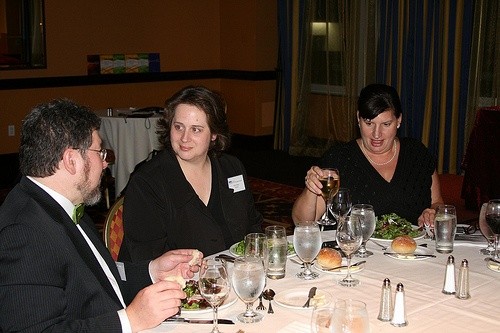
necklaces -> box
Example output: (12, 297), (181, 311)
(361, 139), (396, 164)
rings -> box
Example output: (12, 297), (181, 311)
(305, 179), (309, 182)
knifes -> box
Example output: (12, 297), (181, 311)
(302, 286), (317, 308)
(160, 317), (236, 325)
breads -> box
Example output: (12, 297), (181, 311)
(188, 250), (200, 266)
(316, 248), (342, 268)
(392, 235), (417, 253)
(160, 274), (187, 289)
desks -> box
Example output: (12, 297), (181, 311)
(460, 107), (500, 209)
(136, 231), (500, 333)
(99, 116), (168, 198)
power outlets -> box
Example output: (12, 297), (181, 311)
(8, 125), (15, 136)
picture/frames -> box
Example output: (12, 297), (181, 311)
(0, 0), (47, 69)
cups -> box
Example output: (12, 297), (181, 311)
(434, 205), (458, 254)
(243, 233), (267, 277)
(311, 298), (369, 333)
(263, 225), (287, 279)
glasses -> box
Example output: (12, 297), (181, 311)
(68, 146), (108, 162)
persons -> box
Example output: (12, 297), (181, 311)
(292, 85), (445, 232)
(123, 84), (263, 298)
(0, 99), (202, 333)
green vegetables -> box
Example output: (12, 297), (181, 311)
(369, 213), (421, 239)
(180, 278), (223, 309)
(233, 237), (295, 257)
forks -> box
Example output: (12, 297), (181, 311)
(256, 291), (265, 310)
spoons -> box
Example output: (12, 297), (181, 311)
(263, 287), (276, 314)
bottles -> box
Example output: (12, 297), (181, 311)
(456, 259), (472, 299)
(106, 108), (113, 117)
(377, 277), (394, 322)
(441, 254), (457, 295)
(389, 283), (409, 328)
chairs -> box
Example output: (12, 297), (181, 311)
(104, 196), (124, 264)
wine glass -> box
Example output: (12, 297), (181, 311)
(233, 256), (264, 324)
(294, 221), (323, 279)
(198, 257), (232, 333)
(316, 170), (378, 287)
(480, 199), (500, 273)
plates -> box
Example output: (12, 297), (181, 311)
(160, 275), (238, 313)
(384, 246), (433, 260)
(229, 237), (299, 261)
(273, 286), (332, 310)
(313, 258), (365, 274)
(368, 223), (426, 242)
